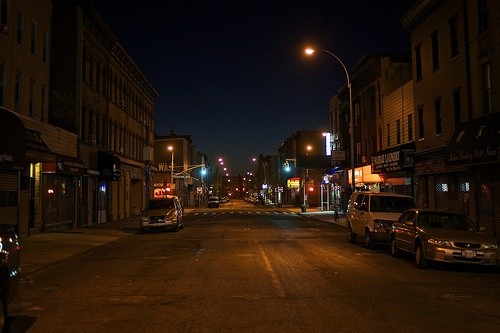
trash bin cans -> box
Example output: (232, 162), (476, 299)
(300, 205), (306, 212)
(278, 202), (283, 208)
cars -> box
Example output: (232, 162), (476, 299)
(0, 223), (25, 333)
(243, 193), (266, 205)
(206, 195), (230, 208)
(390, 208), (500, 270)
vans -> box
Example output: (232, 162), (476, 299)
(345, 191), (418, 250)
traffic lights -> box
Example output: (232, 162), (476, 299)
(200, 166), (207, 177)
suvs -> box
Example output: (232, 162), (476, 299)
(139, 196), (184, 234)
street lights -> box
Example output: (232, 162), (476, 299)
(252, 158), (267, 205)
(167, 145), (174, 195)
(304, 47), (356, 193)
(217, 167), (226, 197)
